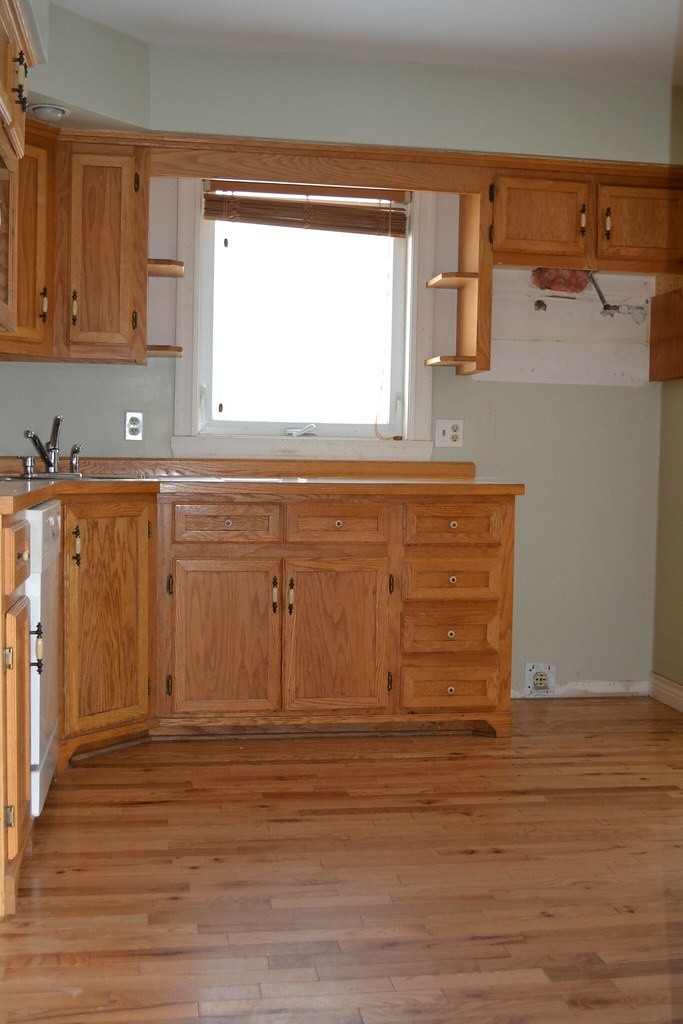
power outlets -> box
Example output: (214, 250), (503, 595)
(436, 419), (462, 446)
(526, 663), (555, 695)
(125, 411), (142, 440)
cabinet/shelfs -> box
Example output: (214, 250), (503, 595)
(0, 517), (32, 917)
(148, 258), (185, 357)
(0, 2), (36, 331)
(61, 494), (149, 771)
(491, 175), (683, 275)
(397, 496), (516, 738)
(0, 136), (148, 364)
(426, 273), (477, 366)
(156, 497), (388, 740)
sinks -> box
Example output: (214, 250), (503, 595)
(1, 475), (63, 481)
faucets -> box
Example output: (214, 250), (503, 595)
(22, 414), (63, 474)
